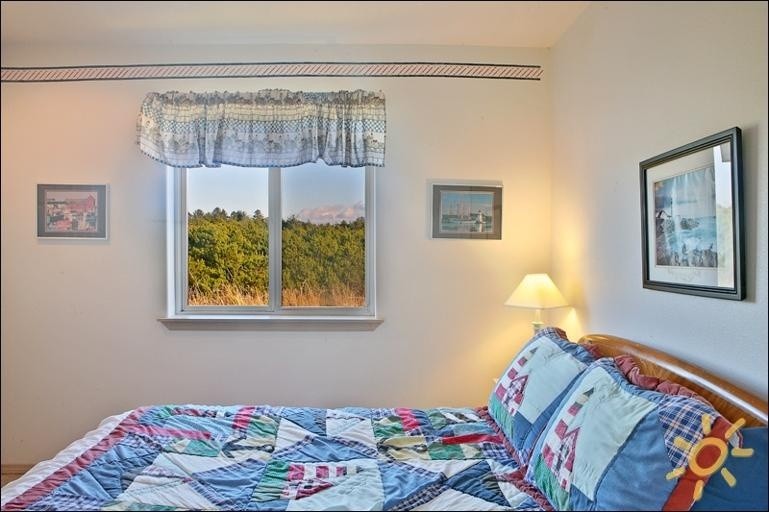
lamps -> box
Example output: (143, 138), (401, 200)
(504, 271), (572, 340)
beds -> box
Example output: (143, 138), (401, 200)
(0, 335), (769, 512)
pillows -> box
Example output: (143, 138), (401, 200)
(503, 354), (740, 511)
(478, 328), (606, 478)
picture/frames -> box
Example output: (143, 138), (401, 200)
(35, 183), (109, 238)
(433, 185), (503, 240)
(638, 127), (745, 302)
